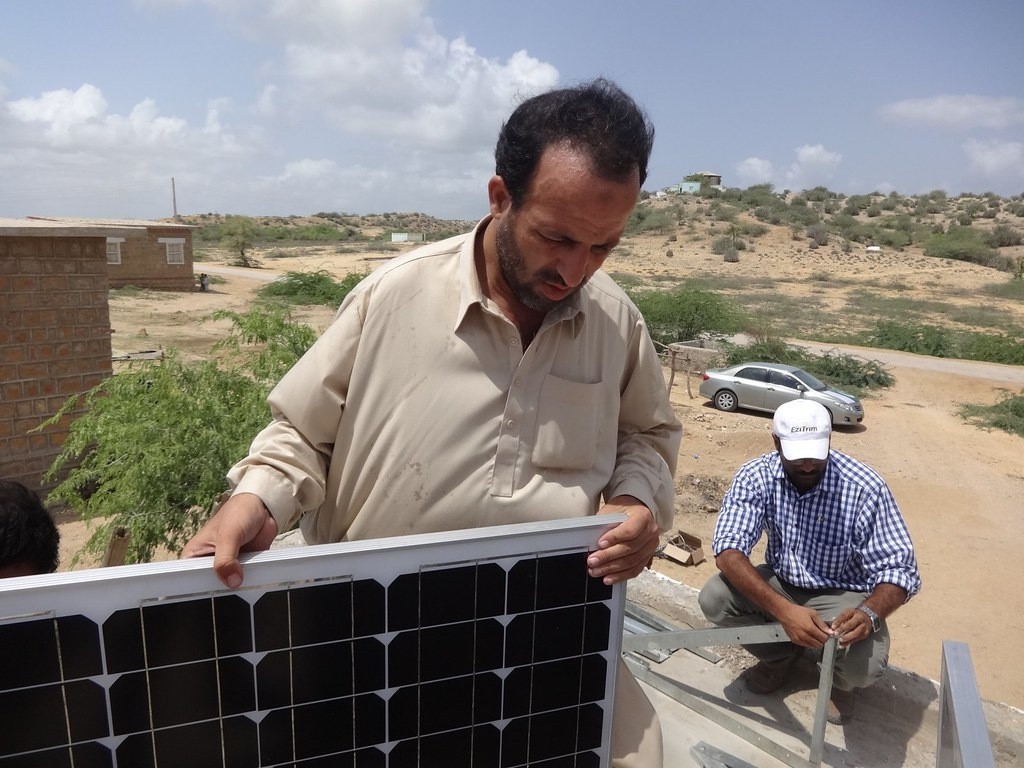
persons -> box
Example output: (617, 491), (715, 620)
(179, 84), (686, 767)
(198, 273), (209, 292)
(698, 398), (924, 723)
(0, 479), (60, 578)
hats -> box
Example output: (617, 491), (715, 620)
(773, 399), (832, 462)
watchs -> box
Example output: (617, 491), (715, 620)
(855, 604), (882, 634)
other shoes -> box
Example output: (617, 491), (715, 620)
(744, 641), (806, 694)
(826, 683), (857, 725)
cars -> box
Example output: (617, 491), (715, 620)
(698, 361), (866, 428)
